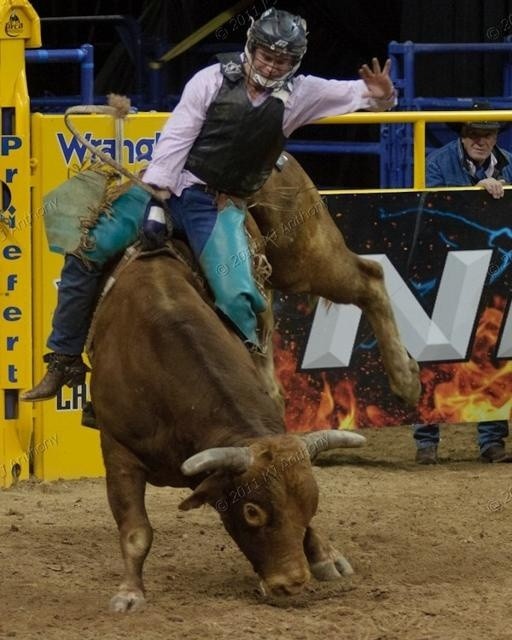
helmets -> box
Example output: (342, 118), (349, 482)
(241, 8), (308, 92)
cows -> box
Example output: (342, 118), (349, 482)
(85, 150), (422, 616)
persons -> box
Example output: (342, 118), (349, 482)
(424, 121), (512, 199)
(23, 9), (398, 401)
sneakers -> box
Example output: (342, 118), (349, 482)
(415, 441), (437, 465)
(478, 444), (509, 464)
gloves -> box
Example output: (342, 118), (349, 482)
(141, 200), (168, 252)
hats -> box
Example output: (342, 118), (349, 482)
(469, 103), (501, 129)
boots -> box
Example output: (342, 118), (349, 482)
(21, 355), (81, 400)
